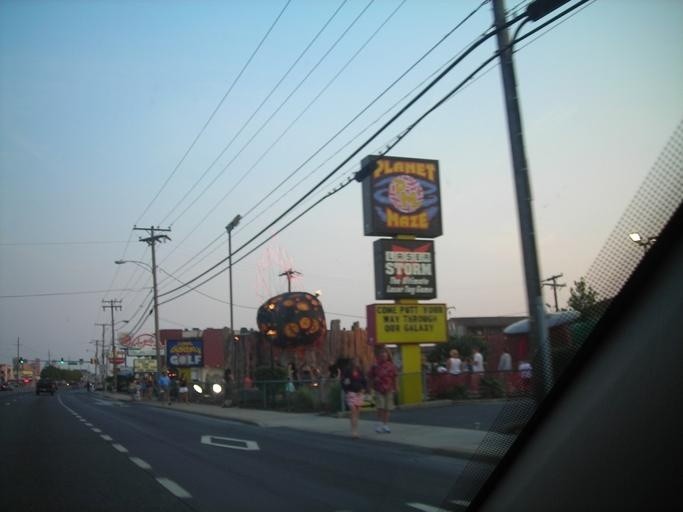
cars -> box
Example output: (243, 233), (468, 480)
(36, 379), (57, 396)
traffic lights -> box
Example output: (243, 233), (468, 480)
(61, 357), (63, 365)
(19, 356), (24, 364)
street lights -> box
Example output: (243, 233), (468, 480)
(114, 258), (162, 374)
(224, 214), (241, 387)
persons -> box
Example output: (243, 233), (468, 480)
(329, 344), (373, 438)
(364, 347), (399, 436)
(134, 365), (296, 410)
(422, 346), (532, 396)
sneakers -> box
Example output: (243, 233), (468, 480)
(376, 425), (391, 433)
